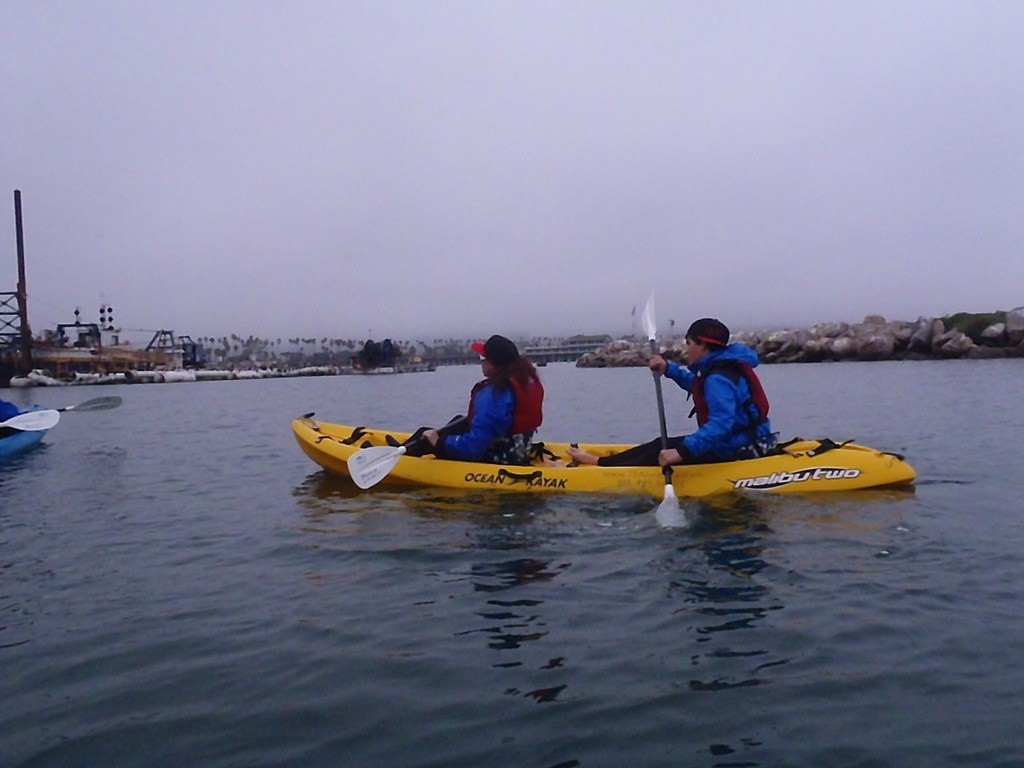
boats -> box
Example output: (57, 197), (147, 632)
(286, 409), (921, 491)
(366, 367), (396, 375)
(141, 329), (183, 370)
(0, 320), (167, 369)
(536, 358), (547, 367)
(0, 402), (55, 462)
(398, 361), (437, 373)
(10, 363), (343, 389)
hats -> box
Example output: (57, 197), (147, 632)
(472, 334), (519, 371)
(687, 318), (731, 352)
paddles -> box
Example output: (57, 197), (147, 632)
(0, 409), (60, 431)
(641, 303), (690, 530)
(346, 416), (467, 490)
(57, 395), (123, 413)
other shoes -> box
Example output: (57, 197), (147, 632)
(386, 435), (401, 448)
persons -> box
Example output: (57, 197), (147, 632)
(360, 334), (535, 465)
(543, 318), (770, 468)
